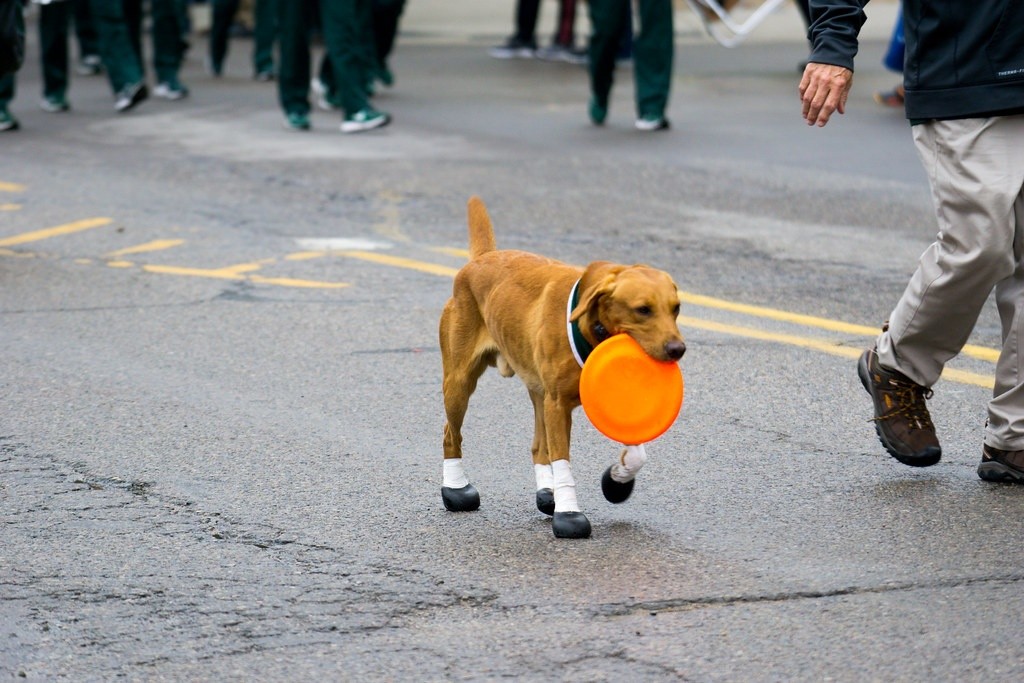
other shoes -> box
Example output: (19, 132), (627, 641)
(115, 84), (146, 112)
(590, 95), (607, 125)
(285, 111), (310, 129)
(378, 67), (394, 87)
(77, 61), (98, 77)
(546, 44), (591, 66)
(0, 107), (17, 132)
(488, 39), (533, 58)
(38, 91), (68, 112)
(873, 82), (906, 107)
(342, 104), (389, 133)
(637, 114), (669, 131)
(154, 79), (186, 101)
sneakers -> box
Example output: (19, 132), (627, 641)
(858, 346), (942, 468)
(975, 440), (1024, 485)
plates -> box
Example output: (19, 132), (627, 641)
(579, 334), (685, 448)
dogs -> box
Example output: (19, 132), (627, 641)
(439, 198), (685, 540)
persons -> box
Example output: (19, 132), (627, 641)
(871, 6), (905, 112)
(589, 0), (673, 130)
(562, 0), (634, 66)
(491, 0), (575, 60)
(799, 0), (1024, 483)
(1, 1), (406, 133)
